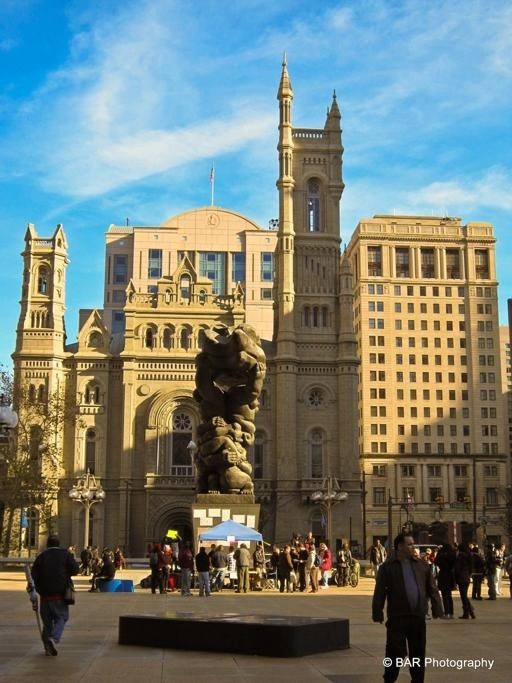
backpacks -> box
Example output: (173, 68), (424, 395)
(315, 554), (321, 567)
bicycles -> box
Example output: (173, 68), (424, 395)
(334, 563), (358, 587)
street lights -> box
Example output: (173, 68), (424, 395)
(0, 407), (19, 464)
(311, 474), (348, 544)
(69, 468), (106, 550)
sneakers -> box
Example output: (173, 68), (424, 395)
(47, 639), (58, 656)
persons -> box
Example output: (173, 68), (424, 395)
(67, 530), (355, 593)
(411, 540), (511, 619)
(369, 537), (386, 575)
(27, 533), (77, 658)
(370, 531), (441, 683)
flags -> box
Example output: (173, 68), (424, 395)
(21, 509), (29, 528)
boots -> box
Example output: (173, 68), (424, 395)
(459, 607), (475, 619)
